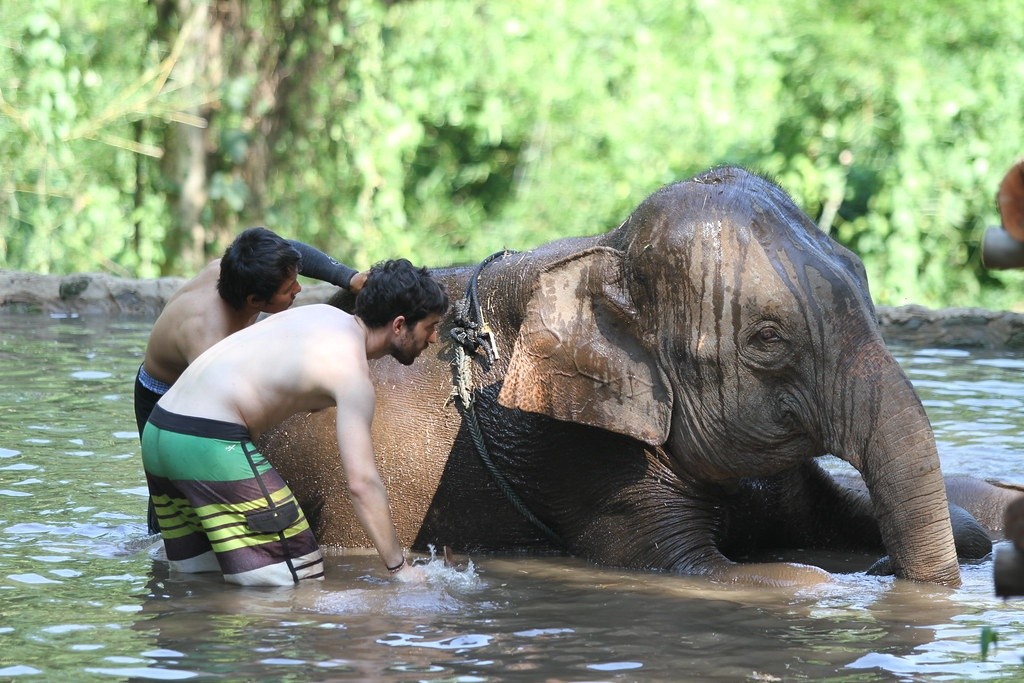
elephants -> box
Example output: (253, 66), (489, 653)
(243, 165), (1022, 604)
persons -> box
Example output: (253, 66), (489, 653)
(134, 228), (380, 537)
(140, 257), (451, 591)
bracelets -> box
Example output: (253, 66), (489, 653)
(386, 555), (408, 573)
